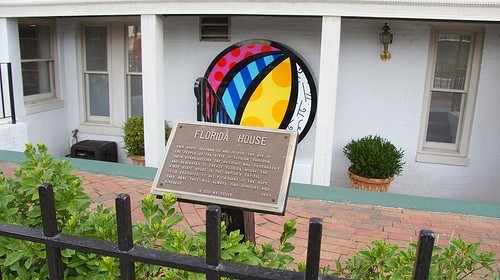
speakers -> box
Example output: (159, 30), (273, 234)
(71, 140), (118, 162)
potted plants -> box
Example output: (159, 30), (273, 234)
(119, 113), (173, 166)
(342, 134), (405, 193)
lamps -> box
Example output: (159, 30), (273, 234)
(378, 22), (393, 63)
